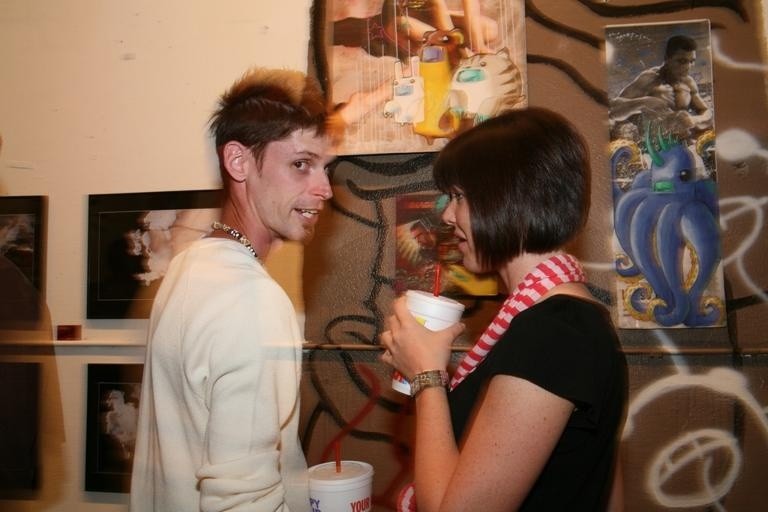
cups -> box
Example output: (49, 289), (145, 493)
(390, 290), (464, 397)
(305, 461), (375, 510)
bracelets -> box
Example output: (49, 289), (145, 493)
(409, 369), (450, 396)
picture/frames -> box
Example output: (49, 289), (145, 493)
(82, 360), (145, 496)
(85, 187), (223, 321)
(0, 193), (51, 330)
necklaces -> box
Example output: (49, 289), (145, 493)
(212, 220), (260, 261)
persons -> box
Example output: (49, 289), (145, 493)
(410, 219), (438, 262)
(128, 63), (340, 512)
(379, 105), (630, 512)
(608, 34), (713, 147)
